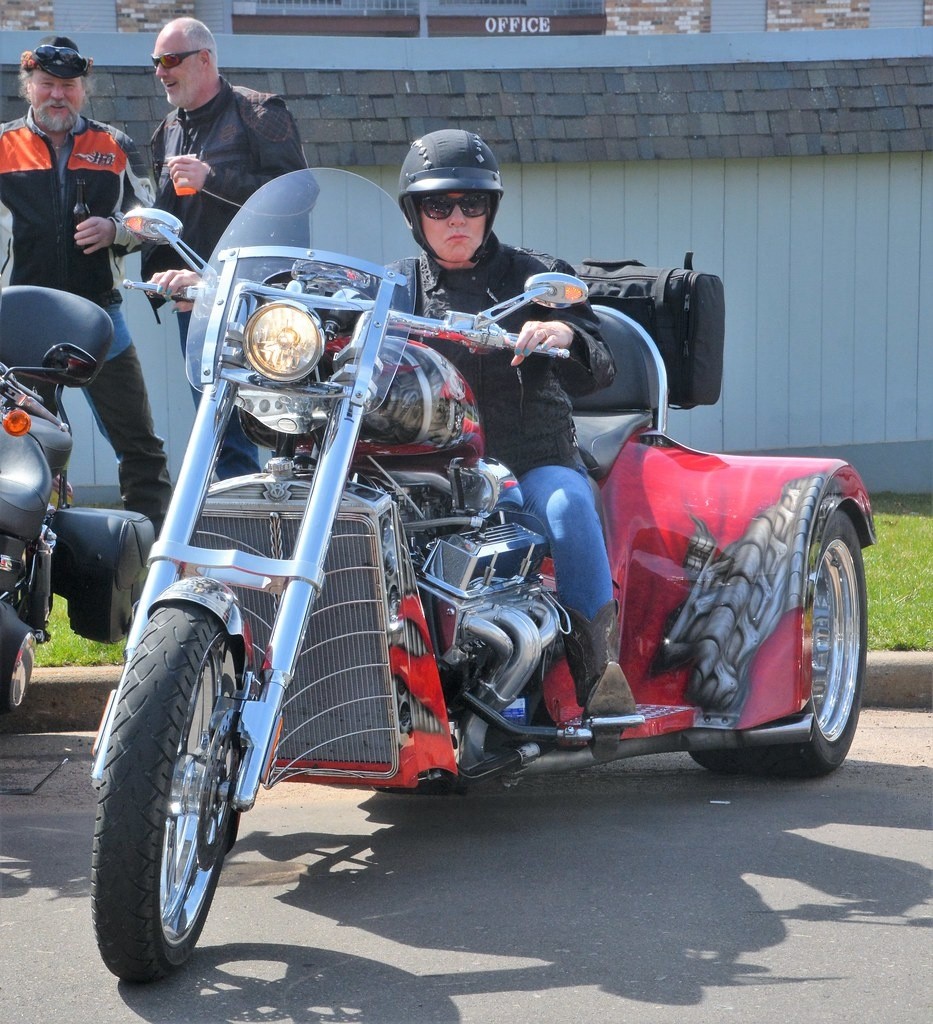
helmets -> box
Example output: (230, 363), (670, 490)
(398, 129), (504, 263)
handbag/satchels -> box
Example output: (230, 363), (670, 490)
(569, 251), (725, 410)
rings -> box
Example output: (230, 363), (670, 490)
(541, 329), (548, 338)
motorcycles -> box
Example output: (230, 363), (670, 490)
(0, 281), (161, 738)
(84, 166), (878, 988)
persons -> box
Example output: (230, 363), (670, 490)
(0, 34), (170, 542)
(141, 17), (319, 481)
(147, 130), (641, 762)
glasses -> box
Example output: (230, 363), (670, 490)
(150, 48), (211, 69)
(418, 193), (491, 219)
(33, 44), (83, 64)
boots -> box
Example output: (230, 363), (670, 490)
(555, 598), (637, 762)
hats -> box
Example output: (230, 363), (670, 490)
(24, 36), (89, 79)
